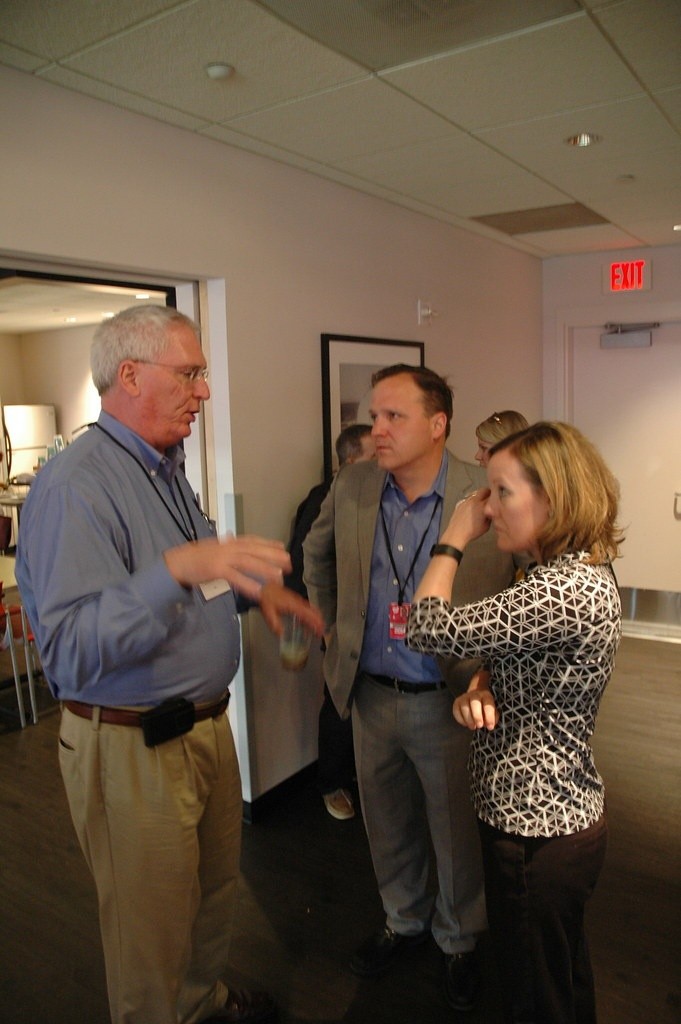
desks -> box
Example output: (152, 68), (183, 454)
(0, 491), (27, 561)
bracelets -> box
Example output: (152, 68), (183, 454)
(430, 543), (464, 567)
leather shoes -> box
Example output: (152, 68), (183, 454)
(360, 925), (429, 973)
(442, 947), (484, 1013)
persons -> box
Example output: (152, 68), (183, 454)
(15, 303), (326, 1024)
(281, 423), (376, 820)
(476, 410), (529, 469)
(302, 362), (534, 1009)
(404, 418), (623, 1023)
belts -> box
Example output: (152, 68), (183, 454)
(369, 674), (448, 692)
(63, 692), (232, 728)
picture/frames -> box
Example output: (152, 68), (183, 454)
(321, 332), (426, 483)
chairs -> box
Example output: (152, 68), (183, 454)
(0, 604), (40, 730)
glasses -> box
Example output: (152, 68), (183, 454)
(135, 359), (210, 384)
(488, 411), (506, 431)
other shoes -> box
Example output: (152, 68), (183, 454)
(321, 786), (356, 821)
(350, 777), (359, 783)
(191, 988), (282, 1024)
